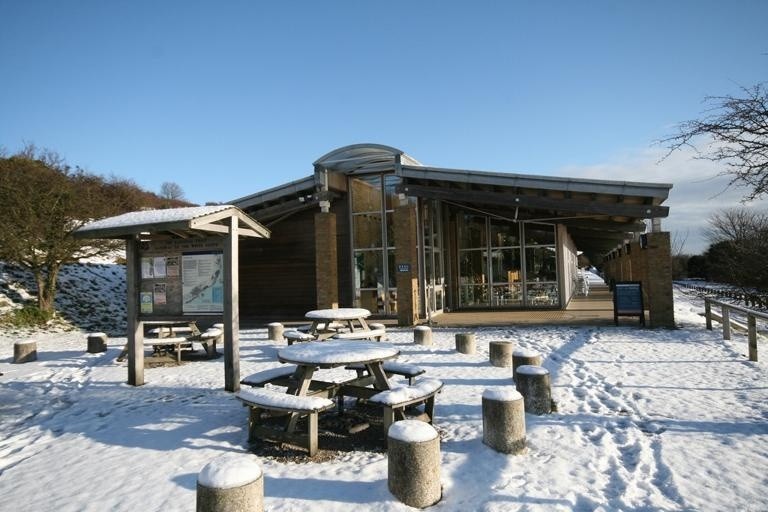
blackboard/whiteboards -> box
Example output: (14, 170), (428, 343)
(613, 281), (644, 316)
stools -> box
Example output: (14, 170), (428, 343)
(387, 419), (442, 507)
(489, 340), (513, 368)
(512, 350), (540, 383)
(13, 339), (38, 363)
(481, 388), (527, 455)
(413, 327), (433, 346)
(455, 333), (476, 354)
(267, 323), (284, 341)
(87, 333), (108, 352)
(193, 452), (264, 511)
(516, 365), (550, 416)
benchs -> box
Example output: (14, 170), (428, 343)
(238, 364), (320, 389)
(345, 360), (426, 386)
(333, 328), (385, 341)
(143, 336), (187, 365)
(369, 377), (445, 435)
(149, 326), (193, 338)
(201, 323), (224, 356)
(234, 387), (336, 456)
(297, 323), (344, 333)
(369, 323), (386, 342)
(343, 322), (386, 330)
(283, 329), (315, 345)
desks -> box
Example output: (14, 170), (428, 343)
(278, 340), (408, 437)
(305, 308), (376, 341)
